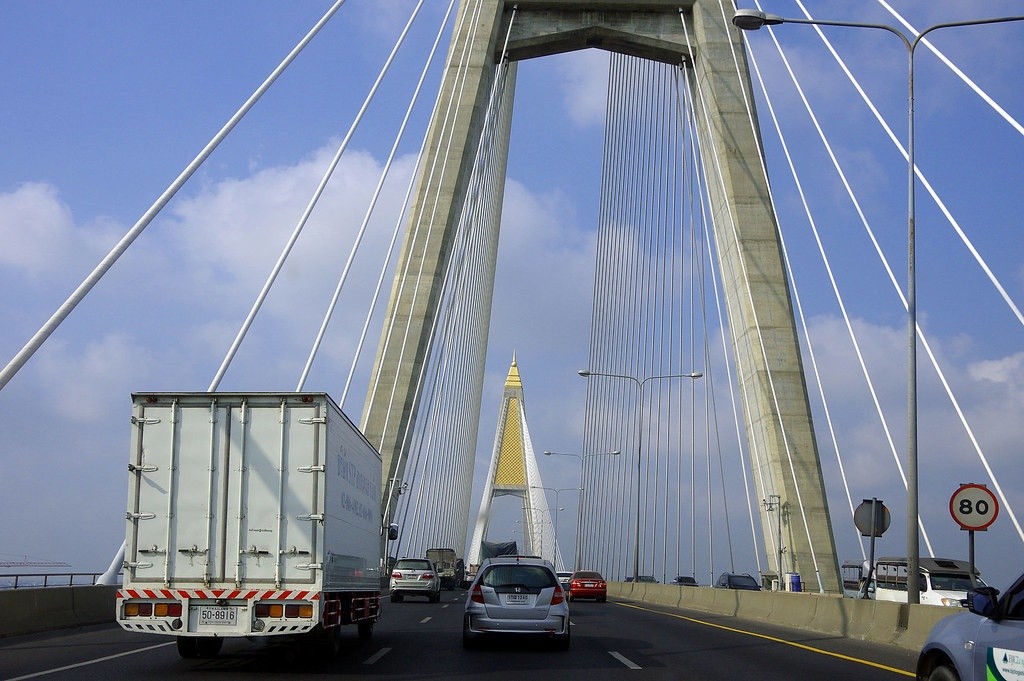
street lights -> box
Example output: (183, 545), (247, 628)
(521, 506), (564, 565)
(575, 369), (703, 581)
(530, 486), (583, 567)
(542, 449), (621, 572)
(731, 6), (1024, 604)
(765, 495), (785, 589)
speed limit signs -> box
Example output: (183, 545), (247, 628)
(949, 484), (1000, 530)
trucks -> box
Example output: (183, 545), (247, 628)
(840, 553), (992, 611)
(425, 547), (457, 592)
(480, 539), (516, 573)
(110, 385), (402, 661)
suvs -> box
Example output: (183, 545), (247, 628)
(712, 573), (762, 593)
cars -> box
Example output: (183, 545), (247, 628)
(567, 569), (607, 603)
(555, 570), (573, 597)
(624, 576), (659, 583)
(461, 555), (571, 651)
(673, 576), (697, 587)
(915, 571), (1024, 681)
(390, 556), (441, 602)
